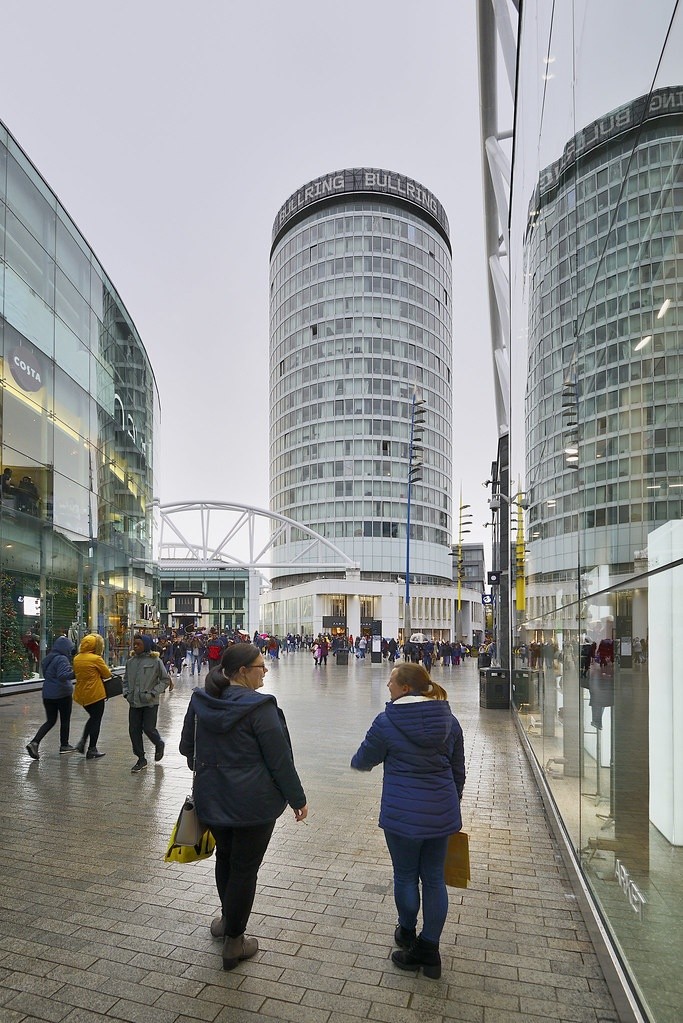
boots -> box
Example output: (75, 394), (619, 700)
(223, 929), (260, 970)
(210, 910), (225, 937)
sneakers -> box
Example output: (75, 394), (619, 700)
(26, 742), (40, 759)
(132, 758), (147, 772)
(155, 741), (164, 761)
(59, 744), (74, 753)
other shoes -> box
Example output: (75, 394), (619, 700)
(86, 746), (106, 759)
(74, 746), (86, 758)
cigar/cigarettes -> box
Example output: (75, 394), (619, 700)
(301, 819), (308, 825)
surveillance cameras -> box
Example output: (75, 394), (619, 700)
(520, 499), (529, 510)
(489, 501), (500, 512)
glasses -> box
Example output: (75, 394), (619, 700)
(248, 664), (265, 668)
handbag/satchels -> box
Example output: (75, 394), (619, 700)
(103, 673), (123, 698)
(262, 647), (265, 652)
(163, 816), (216, 864)
(174, 801), (208, 845)
(443, 832), (470, 889)
(193, 648), (199, 656)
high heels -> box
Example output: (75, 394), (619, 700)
(394, 924), (417, 949)
(391, 936), (441, 979)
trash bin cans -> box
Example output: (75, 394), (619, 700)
(336, 650), (349, 665)
(511, 667), (544, 715)
(480, 666), (510, 709)
(478, 652), (491, 669)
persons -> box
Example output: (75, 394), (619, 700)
(0, 467), (15, 489)
(23, 631), (40, 673)
(281, 633), (401, 665)
(26, 636), (74, 759)
(253, 630), (282, 662)
(520, 637), (648, 677)
(122, 634), (170, 772)
(349, 662), (467, 980)
(149, 624), (252, 678)
(488, 640), (496, 657)
(19, 476), (37, 512)
(402, 637), (480, 675)
(72, 633), (112, 759)
(179, 641), (308, 972)
